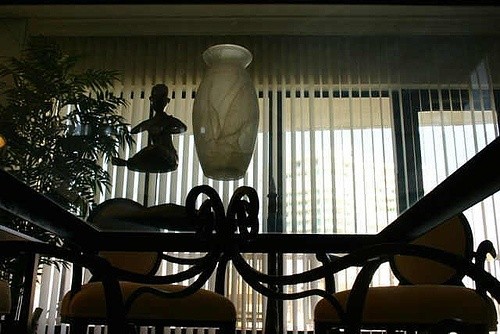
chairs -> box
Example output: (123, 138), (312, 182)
(61, 198), (236, 334)
(313, 211), (500, 334)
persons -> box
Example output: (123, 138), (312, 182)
(127, 81), (189, 174)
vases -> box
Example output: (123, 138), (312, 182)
(192, 44), (260, 181)
